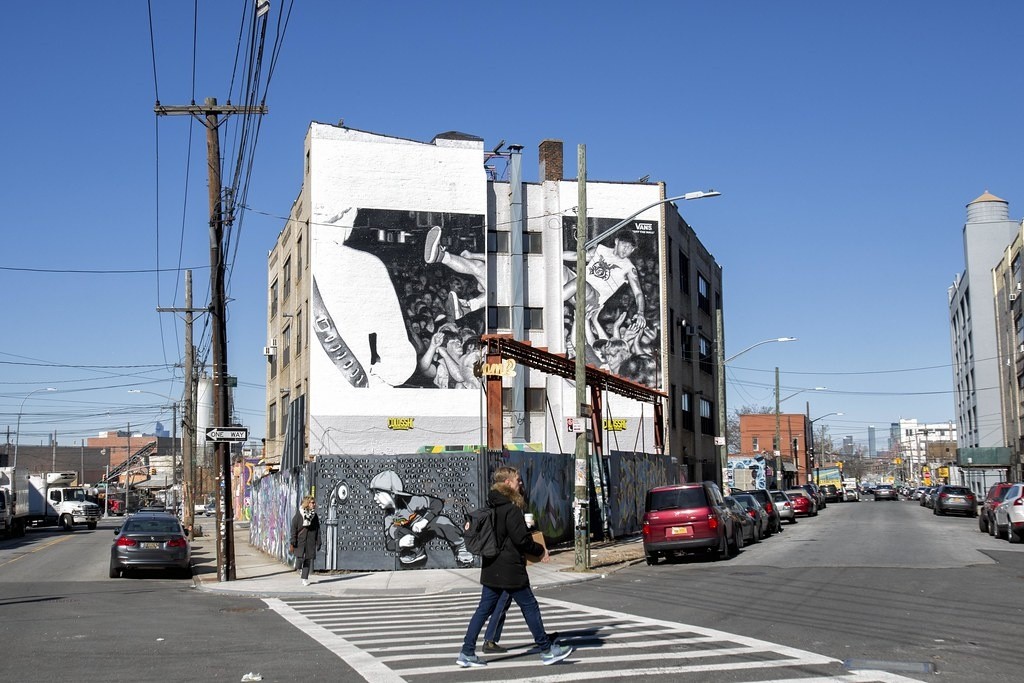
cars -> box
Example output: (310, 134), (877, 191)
(724, 484), (858, 548)
(204, 503), (216, 517)
(933, 485), (978, 518)
(108, 505), (191, 579)
(642, 481), (742, 566)
(861, 483), (938, 509)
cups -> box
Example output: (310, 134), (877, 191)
(524, 513), (533, 528)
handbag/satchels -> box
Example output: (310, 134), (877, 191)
(532, 531), (550, 563)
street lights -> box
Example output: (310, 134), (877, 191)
(573, 190), (720, 569)
(775, 388), (828, 491)
(127, 391), (185, 523)
(807, 413), (845, 485)
(822, 437), (854, 468)
(11, 388), (58, 516)
(717, 338), (797, 497)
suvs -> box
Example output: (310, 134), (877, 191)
(977, 482), (1024, 544)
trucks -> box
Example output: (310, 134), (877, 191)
(0, 466), (126, 541)
(166, 500), (207, 515)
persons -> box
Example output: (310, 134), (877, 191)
(290, 496), (322, 586)
(456, 467), (573, 667)
(311, 204), (663, 391)
(139, 498), (144, 506)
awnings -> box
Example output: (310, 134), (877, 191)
(782, 462), (798, 471)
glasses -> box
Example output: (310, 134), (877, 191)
(309, 502), (315, 504)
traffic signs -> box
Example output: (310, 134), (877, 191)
(580, 404), (593, 418)
(206, 427), (248, 442)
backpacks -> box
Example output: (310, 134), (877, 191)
(464, 505), (500, 556)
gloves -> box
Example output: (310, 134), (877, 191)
(525, 513), (536, 529)
(290, 544), (294, 553)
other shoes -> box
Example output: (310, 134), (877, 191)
(303, 578), (310, 586)
(300, 569), (303, 574)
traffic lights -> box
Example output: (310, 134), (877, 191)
(149, 469), (156, 476)
(103, 474), (106, 481)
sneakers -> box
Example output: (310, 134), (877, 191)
(456, 653), (487, 667)
(540, 644), (573, 665)
(547, 632), (559, 644)
(483, 641), (508, 653)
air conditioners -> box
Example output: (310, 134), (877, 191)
(264, 347), (276, 356)
(1009, 294), (1016, 301)
